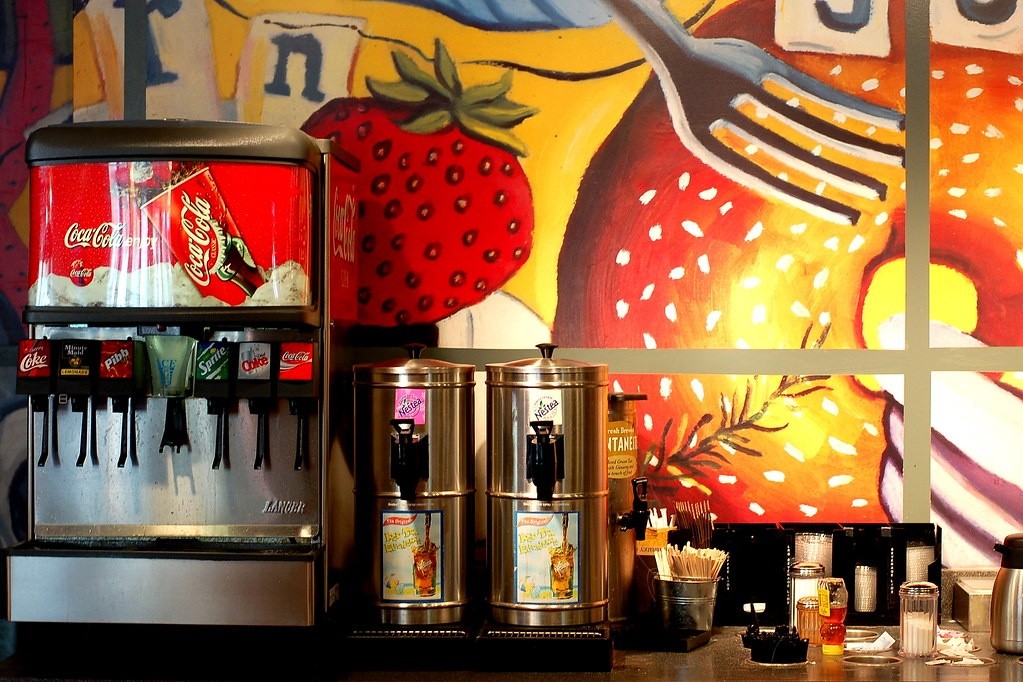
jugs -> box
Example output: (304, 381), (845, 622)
(989, 533), (1023, 655)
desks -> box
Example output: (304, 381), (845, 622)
(350, 625), (1023, 682)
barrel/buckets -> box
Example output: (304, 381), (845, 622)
(608, 395), (650, 628)
(365, 342), (477, 625)
(485, 343), (609, 627)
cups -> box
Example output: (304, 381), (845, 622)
(654, 576), (720, 632)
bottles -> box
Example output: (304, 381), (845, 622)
(788, 560), (827, 634)
(796, 596), (822, 647)
(898, 581), (940, 658)
(817, 577), (848, 656)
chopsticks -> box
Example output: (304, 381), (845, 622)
(654, 541), (729, 582)
